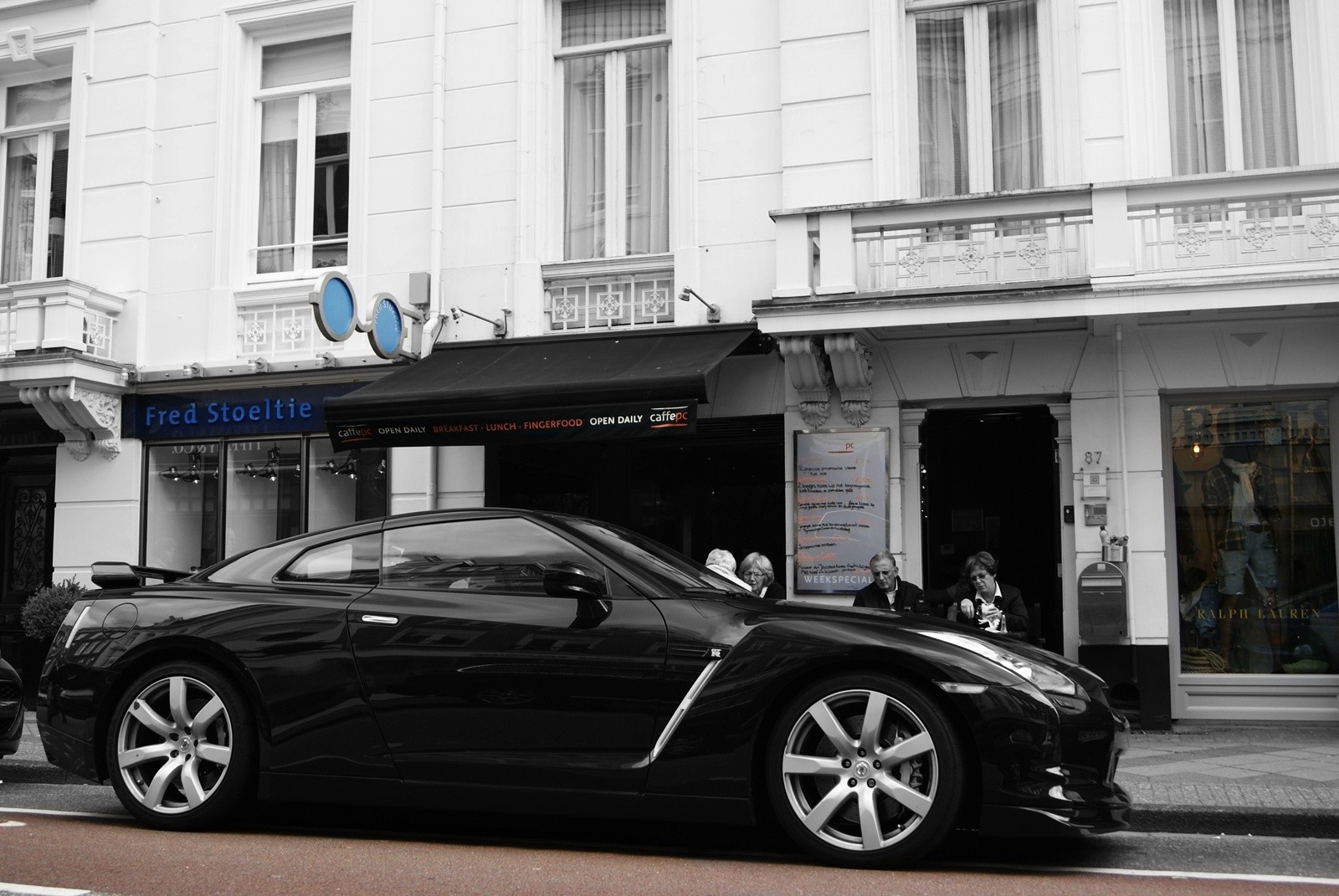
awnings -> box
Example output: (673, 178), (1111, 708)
(321, 322), (763, 455)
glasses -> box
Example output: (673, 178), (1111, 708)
(743, 572), (765, 579)
(970, 571), (988, 581)
(873, 570), (890, 576)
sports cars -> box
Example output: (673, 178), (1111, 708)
(33, 506), (1134, 867)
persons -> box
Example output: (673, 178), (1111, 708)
(1200, 457), (1289, 675)
(737, 551), (787, 599)
(954, 550), (1032, 635)
(698, 548), (753, 595)
(848, 551), (926, 613)
